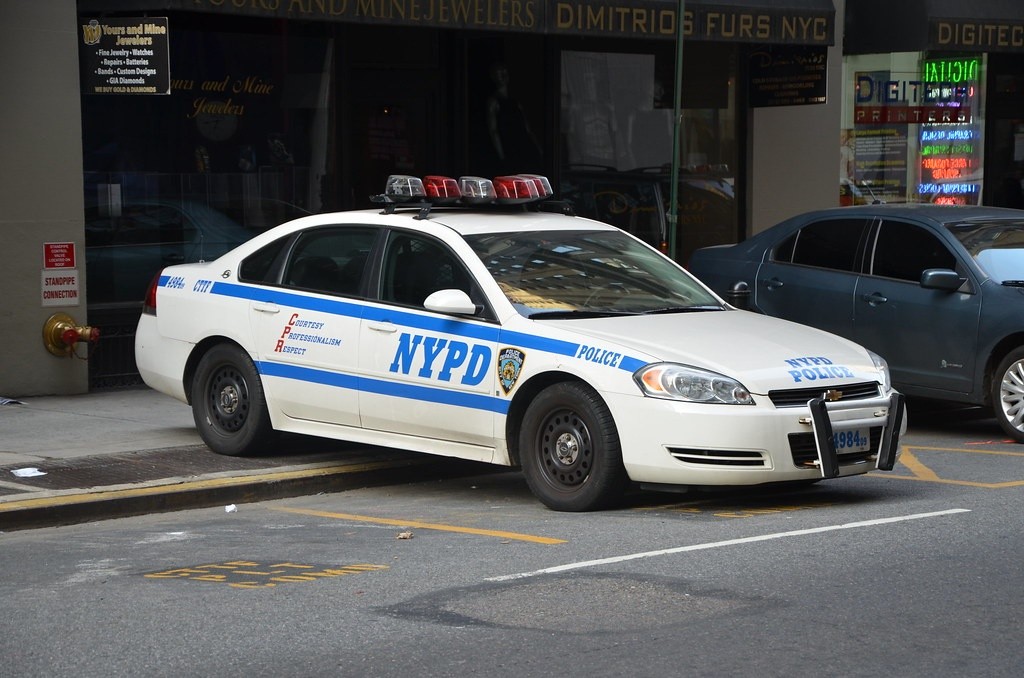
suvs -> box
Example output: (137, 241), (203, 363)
(549, 163), (692, 251)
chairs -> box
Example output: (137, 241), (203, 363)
(288, 252), (369, 296)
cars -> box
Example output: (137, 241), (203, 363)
(85, 195), (371, 327)
(133, 173), (909, 513)
(689, 204), (1023, 445)
